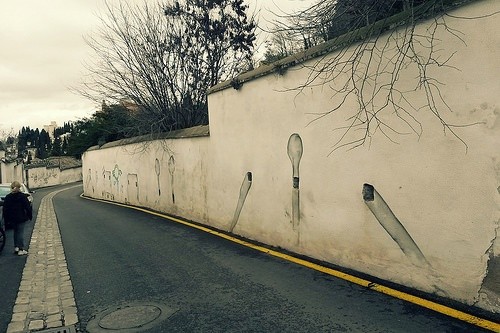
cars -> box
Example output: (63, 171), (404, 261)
(0, 182), (35, 208)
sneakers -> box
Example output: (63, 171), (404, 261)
(18, 249), (27, 255)
(14, 246), (19, 252)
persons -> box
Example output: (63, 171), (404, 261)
(2, 180), (33, 256)
